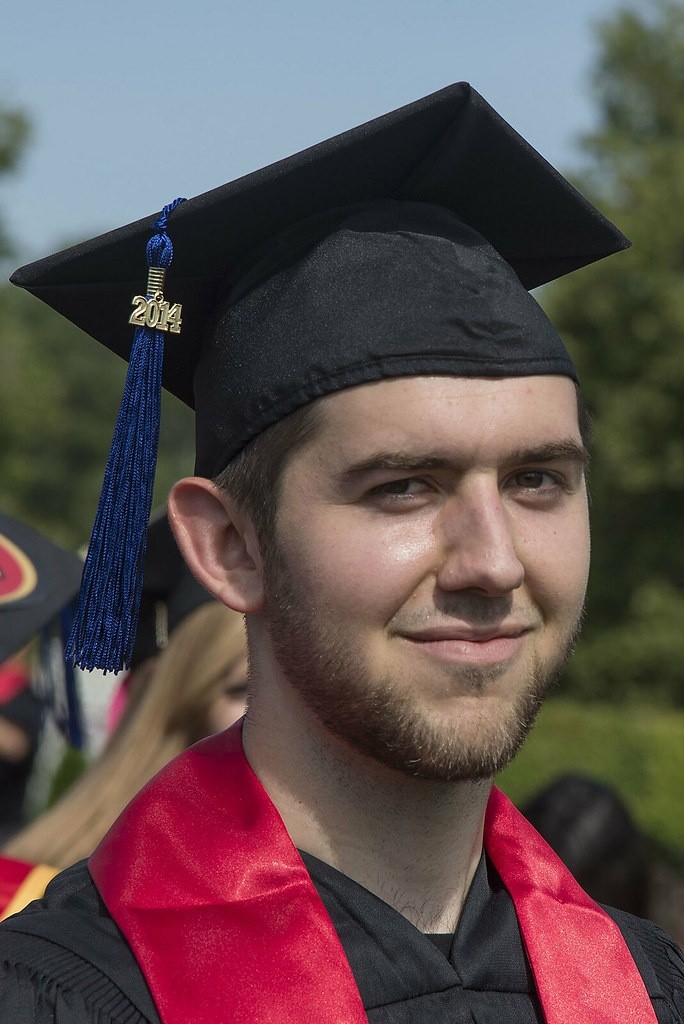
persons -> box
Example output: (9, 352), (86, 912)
(0, 509), (249, 919)
(0, 78), (683, 1024)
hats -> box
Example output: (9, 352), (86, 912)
(38, 511), (220, 749)
(0, 514), (87, 672)
(9, 82), (634, 676)
